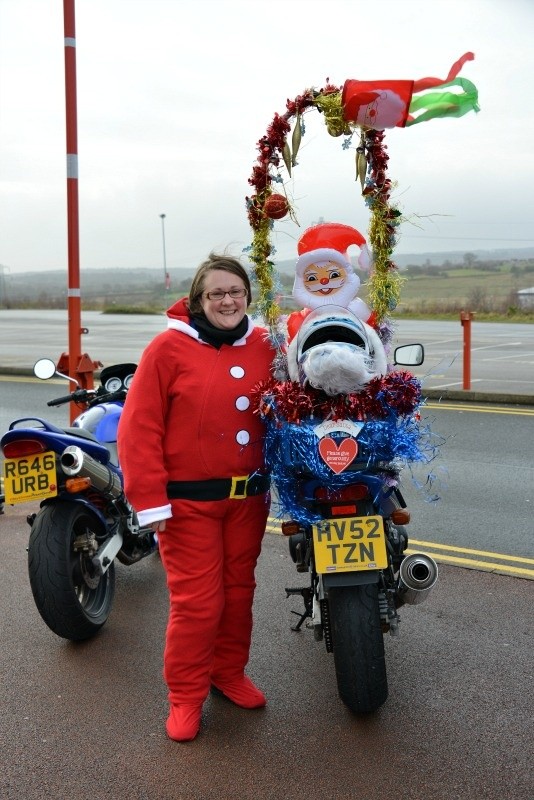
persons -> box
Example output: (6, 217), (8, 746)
(117, 253), (277, 741)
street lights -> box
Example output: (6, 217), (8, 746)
(160, 213), (168, 304)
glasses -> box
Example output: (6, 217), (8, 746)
(201, 289), (249, 300)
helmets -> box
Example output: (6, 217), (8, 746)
(296, 315), (377, 390)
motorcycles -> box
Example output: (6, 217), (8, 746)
(0, 357), (160, 641)
(281, 343), (439, 718)
